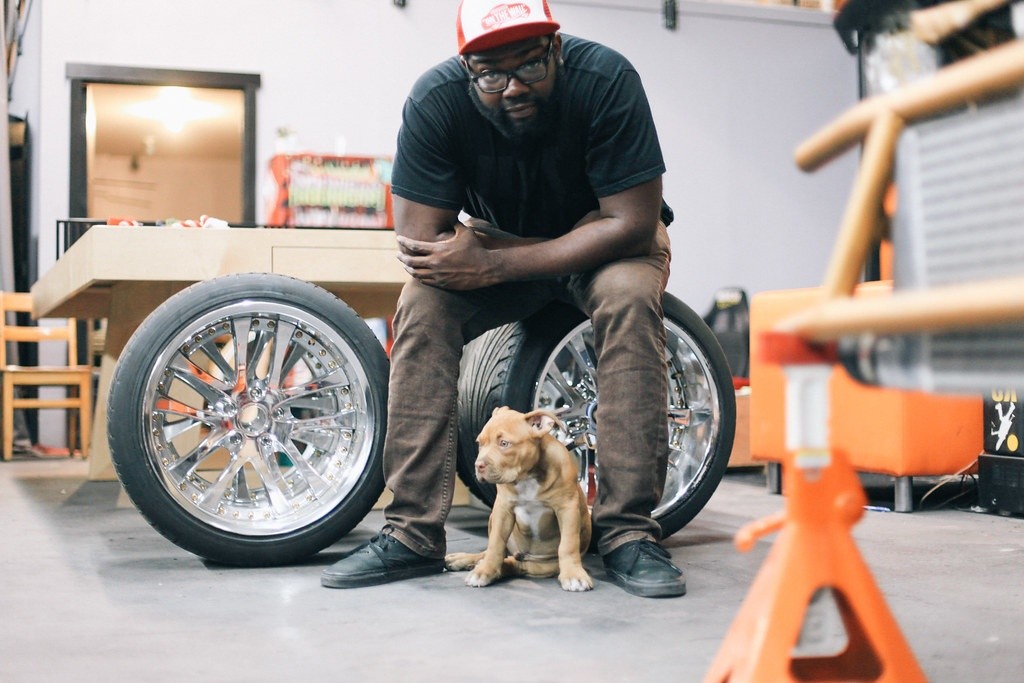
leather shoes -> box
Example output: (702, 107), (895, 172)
(603, 539), (686, 597)
(320, 523), (446, 589)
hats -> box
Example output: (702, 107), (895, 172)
(456, 0), (560, 54)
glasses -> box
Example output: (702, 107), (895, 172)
(465, 34), (552, 94)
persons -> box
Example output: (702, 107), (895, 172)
(320, 0), (686, 597)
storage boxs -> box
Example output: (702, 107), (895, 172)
(977, 382), (1024, 518)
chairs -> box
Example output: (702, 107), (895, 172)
(0, 290), (95, 462)
(749, 277), (984, 515)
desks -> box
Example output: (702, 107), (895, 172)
(30, 224), (416, 482)
